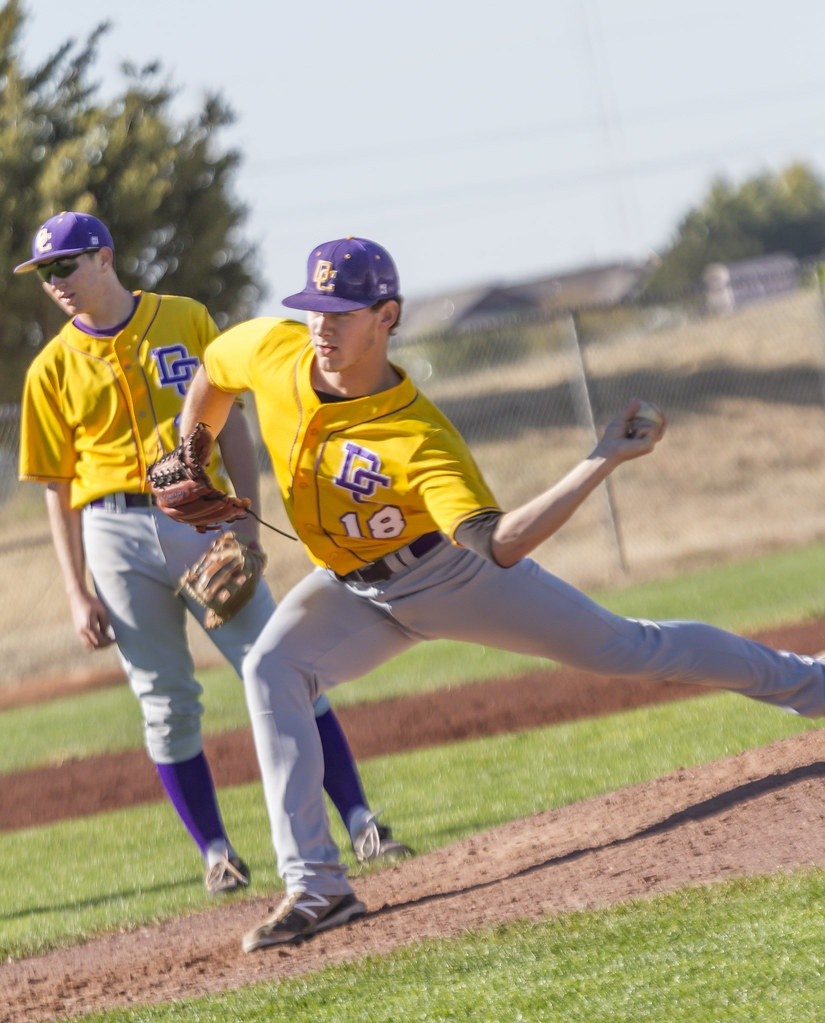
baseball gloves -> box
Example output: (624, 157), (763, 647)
(146, 427), (252, 534)
(179, 530), (269, 631)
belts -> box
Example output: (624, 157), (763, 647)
(91, 493), (157, 507)
(334, 530), (443, 584)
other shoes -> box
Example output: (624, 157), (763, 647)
(204, 848), (250, 893)
(351, 807), (415, 866)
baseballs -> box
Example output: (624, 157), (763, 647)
(629, 399), (662, 433)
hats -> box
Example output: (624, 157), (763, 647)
(14, 211), (114, 272)
(281, 236), (399, 314)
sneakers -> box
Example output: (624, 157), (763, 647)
(242, 891), (367, 954)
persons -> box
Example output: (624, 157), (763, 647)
(177, 239), (825, 956)
(12, 210), (418, 893)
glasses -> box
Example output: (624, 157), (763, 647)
(35, 249), (100, 283)
